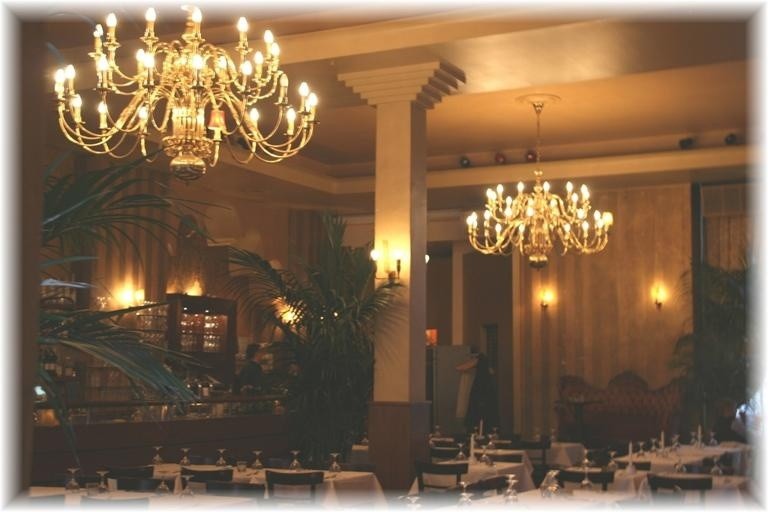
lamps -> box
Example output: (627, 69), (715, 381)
(465, 94), (613, 269)
(51, 5), (320, 187)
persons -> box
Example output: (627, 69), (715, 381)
(232, 343), (266, 391)
(703, 395), (741, 475)
(454, 344), (499, 436)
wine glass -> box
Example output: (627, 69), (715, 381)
(62, 446), (343, 501)
(396, 425), (725, 504)
